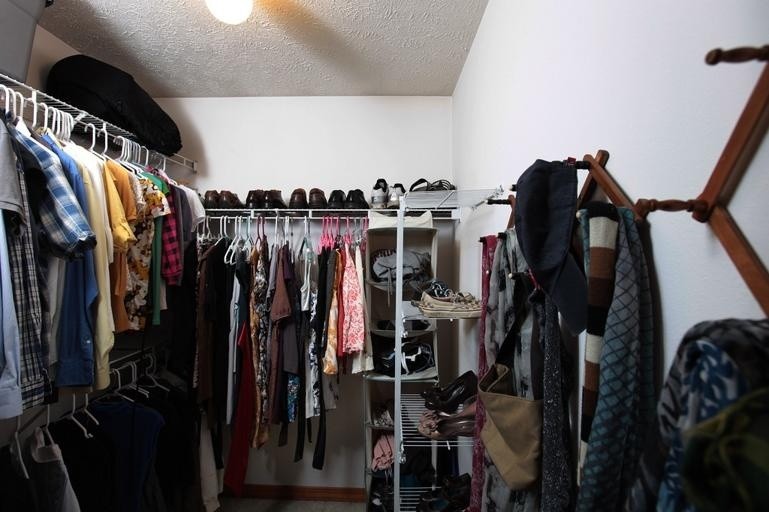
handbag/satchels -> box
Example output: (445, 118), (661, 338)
(44, 55), (183, 157)
(473, 360), (544, 496)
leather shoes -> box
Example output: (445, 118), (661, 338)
(416, 473), (470, 512)
(416, 370), (478, 441)
(205, 188), (370, 209)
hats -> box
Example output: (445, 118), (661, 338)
(512, 159), (592, 341)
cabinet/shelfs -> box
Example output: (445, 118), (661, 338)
(366, 185), (504, 512)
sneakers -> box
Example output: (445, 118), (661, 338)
(371, 178), (405, 210)
(411, 281), (482, 319)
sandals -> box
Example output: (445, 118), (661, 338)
(409, 177), (455, 191)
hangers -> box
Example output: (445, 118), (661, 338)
(8, 353), (169, 479)
(0, 84), (172, 181)
(197, 214), (367, 267)
